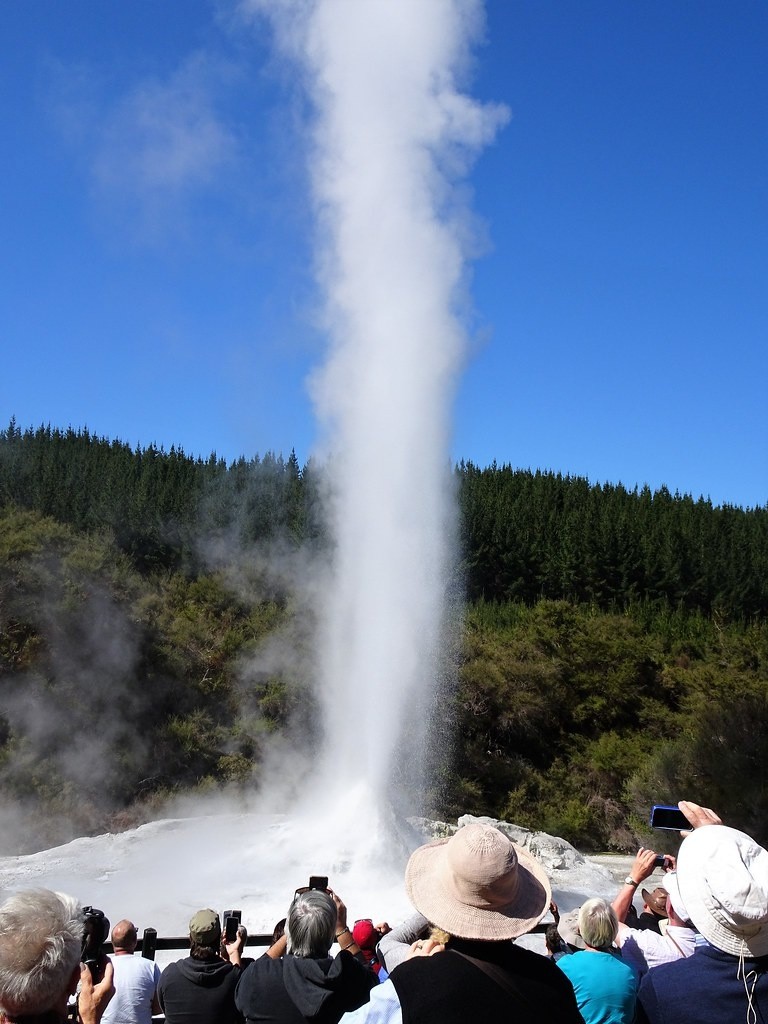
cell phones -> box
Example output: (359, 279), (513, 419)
(310, 877), (328, 893)
(226, 910), (241, 941)
(651, 805), (694, 832)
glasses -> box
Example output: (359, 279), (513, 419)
(354, 919), (372, 925)
(133, 927), (139, 934)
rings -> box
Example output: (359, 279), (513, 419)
(416, 945), (422, 949)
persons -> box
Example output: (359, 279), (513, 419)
(157, 907), (243, 1024)
(609, 846), (708, 975)
(374, 909), (433, 976)
(235, 886), (380, 1024)
(1, 885), (117, 1024)
(338, 825), (587, 1024)
(555, 897), (638, 1024)
(220, 924), (256, 971)
(544, 887), (667, 965)
(638, 801), (768, 1024)
(352, 918), (392, 982)
(99, 920), (164, 1024)
(270, 918), (287, 947)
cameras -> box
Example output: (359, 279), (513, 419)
(81, 906), (110, 985)
(653, 856), (669, 868)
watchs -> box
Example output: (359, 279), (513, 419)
(624, 876), (639, 888)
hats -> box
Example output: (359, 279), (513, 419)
(642, 888), (670, 918)
(406, 825), (552, 940)
(557, 908), (588, 949)
(352, 921), (378, 948)
(190, 908), (220, 944)
(662, 869), (689, 923)
(676, 825), (768, 958)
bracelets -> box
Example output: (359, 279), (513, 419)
(335, 927), (349, 939)
(341, 941), (356, 952)
